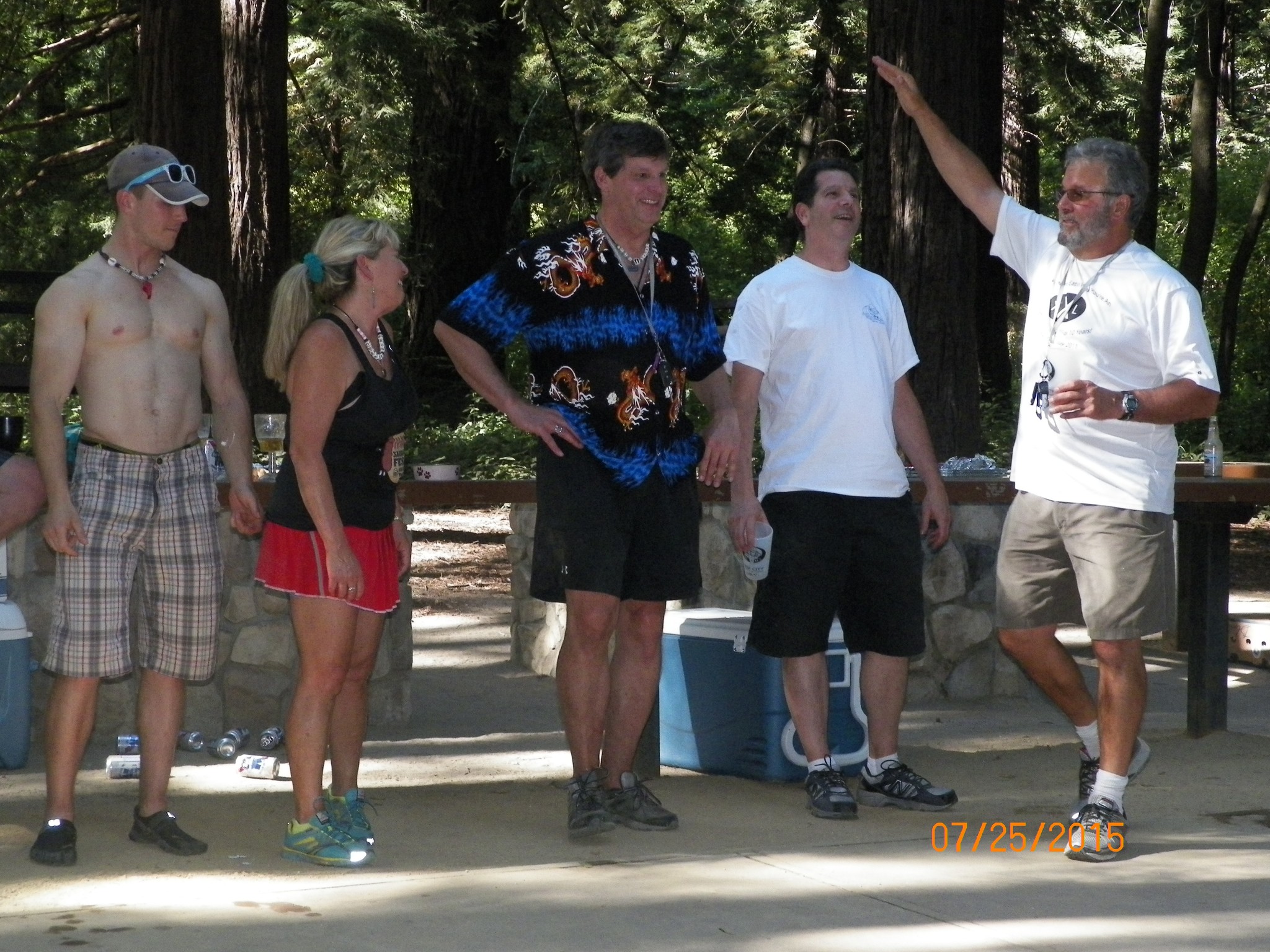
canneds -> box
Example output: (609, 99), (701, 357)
(206, 724), (284, 759)
(178, 731), (204, 751)
(204, 438), (227, 480)
(235, 754), (280, 779)
(116, 735), (140, 754)
(106, 755), (140, 779)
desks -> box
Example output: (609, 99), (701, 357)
(218, 462), (1270, 739)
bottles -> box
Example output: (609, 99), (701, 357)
(1204, 416), (1223, 478)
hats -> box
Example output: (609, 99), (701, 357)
(107, 143), (209, 206)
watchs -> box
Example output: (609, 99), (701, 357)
(1119, 389), (1138, 422)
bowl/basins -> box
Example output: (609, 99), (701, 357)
(413, 464), (460, 481)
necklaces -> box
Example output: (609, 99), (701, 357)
(326, 296), (388, 376)
(97, 245), (167, 300)
(600, 220), (651, 295)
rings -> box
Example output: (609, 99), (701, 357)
(553, 424), (563, 436)
(346, 586), (356, 592)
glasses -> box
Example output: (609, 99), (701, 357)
(1058, 189), (1134, 202)
(124, 163), (196, 191)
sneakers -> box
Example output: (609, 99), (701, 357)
(280, 797), (375, 867)
(856, 759), (958, 811)
(804, 754), (858, 819)
(566, 767), (617, 839)
(598, 771), (679, 832)
(1064, 795), (1128, 863)
(326, 783), (379, 846)
(1068, 736), (1153, 829)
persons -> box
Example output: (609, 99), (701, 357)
(256, 214), (410, 868)
(433, 109), (739, 838)
(0, 143), (265, 864)
(872, 54), (1221, 862)
(730, 155), (953, 822)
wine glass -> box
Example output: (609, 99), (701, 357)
(254, 413), (286, 483)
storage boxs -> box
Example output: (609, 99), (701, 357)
(660, 608), (869, 783)
(0, 599), (33, 770)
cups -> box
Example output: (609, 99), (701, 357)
(1046, 348), (1081, 409)
(0, 416), (24, 455)
(198, 414), (211, 449)
(743, 520), (773, 581)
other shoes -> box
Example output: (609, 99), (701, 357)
(29, 818), (78, 867)
(128, 804), (208, 855)
